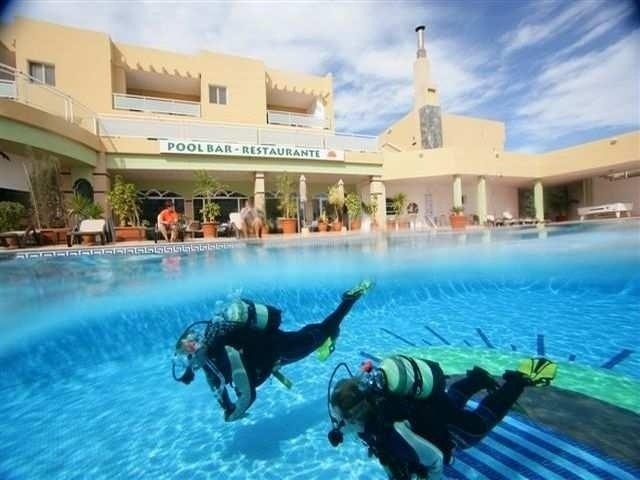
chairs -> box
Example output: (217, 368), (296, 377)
(154, 214), (187, 243)
(227, 212), (262, 239)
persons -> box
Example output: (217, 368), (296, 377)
(241, 200), (264, 240)
(169, 280), (374, 421)
(157, 201), (180, 243)
(159, 254), (183, 275)
(329, 353), (559, 479)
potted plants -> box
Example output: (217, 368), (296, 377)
(62, 173), (149, 246)
(0, 200), (26, 249)
(190, 168), (230, 239)
(270, 170), (410, 234)
(448, 205), (468, 229)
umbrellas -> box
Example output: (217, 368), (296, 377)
(337, 178), (347, 227)
(298, 173), (308, 227)
